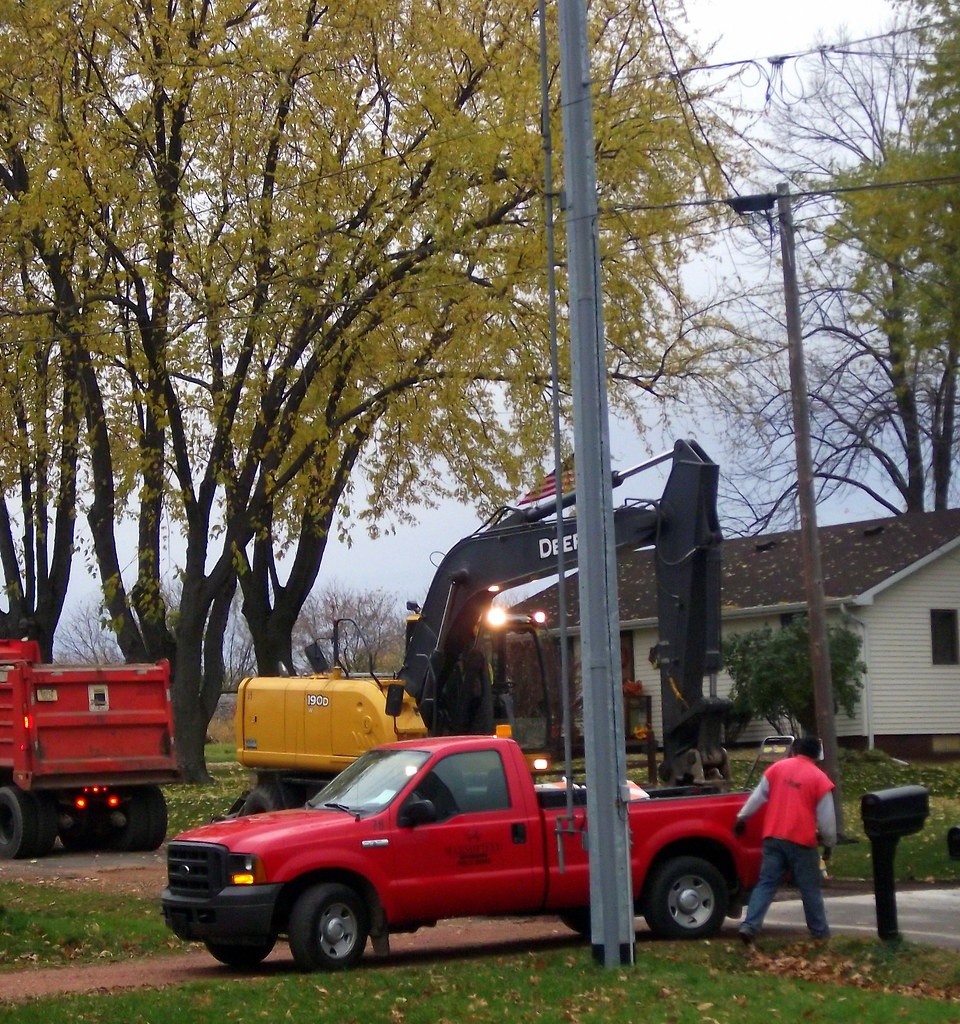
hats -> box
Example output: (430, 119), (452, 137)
(791, 738), (821, 758)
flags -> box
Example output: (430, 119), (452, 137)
(517, 451), (575, 506)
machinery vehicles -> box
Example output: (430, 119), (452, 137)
(226, 441), (751, 817)
(552, 674), (657, 762)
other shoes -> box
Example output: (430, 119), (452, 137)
(739, 925), (753, 944)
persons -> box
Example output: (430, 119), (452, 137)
(733, 737), (838, 947)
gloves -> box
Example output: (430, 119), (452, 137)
(822, 847), (832, 862)
(733, 820), (744, 837)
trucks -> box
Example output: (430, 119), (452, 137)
(0, 635), (187, 863)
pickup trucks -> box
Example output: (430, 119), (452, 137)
(159, 725), (795, 975)
